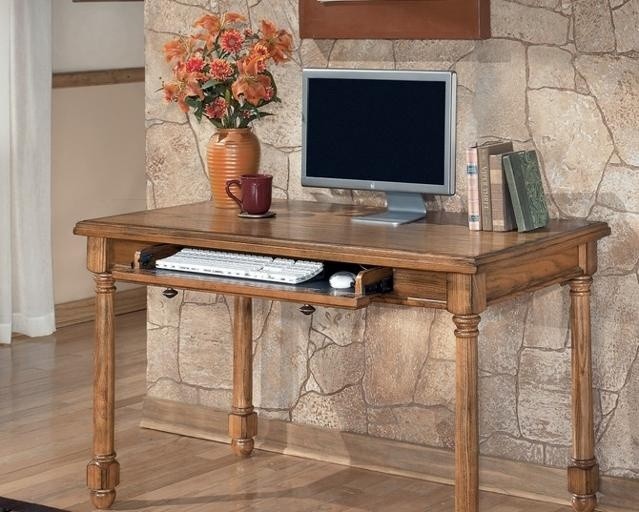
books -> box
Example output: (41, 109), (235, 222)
(467, 140), (549, 233)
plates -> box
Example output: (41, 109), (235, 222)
(237, 212), (275, 218)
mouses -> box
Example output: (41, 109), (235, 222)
(329, 270), (357, 288)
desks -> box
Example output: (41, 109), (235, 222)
(72, 198), (612, 512)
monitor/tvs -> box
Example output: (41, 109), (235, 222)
(300, 67), (458, 226)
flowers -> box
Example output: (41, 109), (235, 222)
(162, 13), (293, 128)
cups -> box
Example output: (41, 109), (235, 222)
(225, 174), (273, 215)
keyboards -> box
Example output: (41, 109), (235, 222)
(155, 248), (324, 285)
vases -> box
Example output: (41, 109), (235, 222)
(208, 128), (261, 208)
